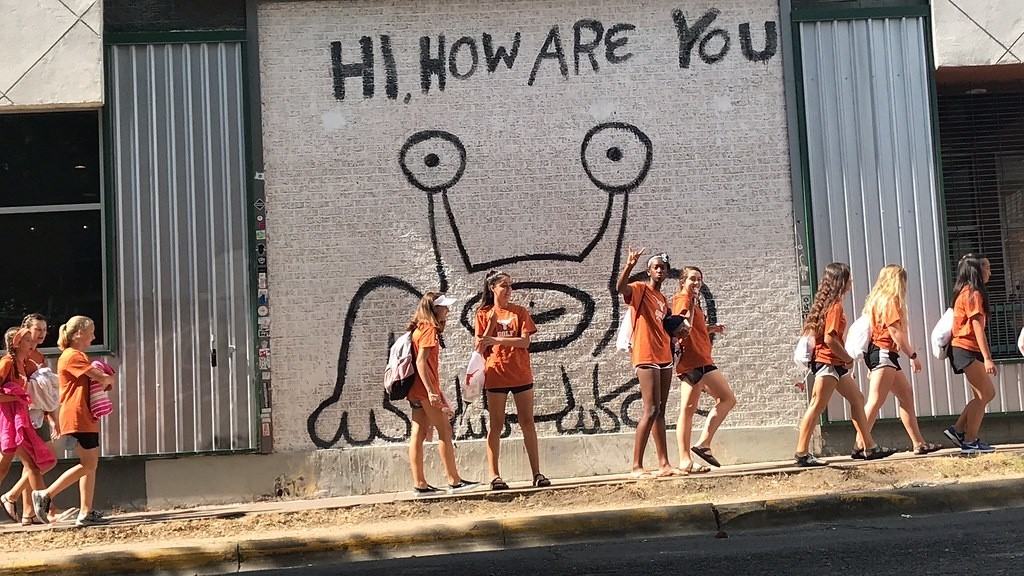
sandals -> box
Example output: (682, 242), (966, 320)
(22, 502), (56, 526)
(851, 442), (864, 459)
(0, 495), (18, 522)
(533, 474), (550, 486)
(491, 477), (509, 490)
(864, 445), (898, 460)
(794, 452), (829, 466)
(914, 440), (944, 454)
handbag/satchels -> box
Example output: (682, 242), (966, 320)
(463, 342), (486, 403)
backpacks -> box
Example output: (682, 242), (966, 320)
(931, 286), (972, 360)
(383, 325), (446, 401)
(616, 282), (646, 354)
(845, 291), (882, 361)
(672, 297), (694, 366)
(793, 329), (816, 376)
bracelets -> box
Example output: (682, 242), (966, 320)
(909, 352), (917, 360)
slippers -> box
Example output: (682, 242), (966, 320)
(628, 469), (657, 479)
(657, 466), (689, 476)
(691, 446), (720, 467)
(676, 461), (711, 474)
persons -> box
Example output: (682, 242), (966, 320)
(407, 291), (482, 497)
(32, 315), (115, 527)
(1018, 326), (1024, 356)
(1, 313), (80, 522)
(616, 244), (689, 481)
(0, 326), (70, 524)
(670, 266), (736, 475)
(943, 252), (997, 454)
(475, 270), (551, 491)
(852, 266), (941, 461)
(794, 264), (899, 467)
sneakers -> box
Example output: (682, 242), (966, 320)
(32, 490), (50, 524)
(944, 425), (966, 447)
(414, 484), (447, 497)
(447, 479), (480, 494)
(961, 438), (996, 455)
(76, 510), (111, 527)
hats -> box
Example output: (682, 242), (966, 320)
(433, 295), (457, 309)
